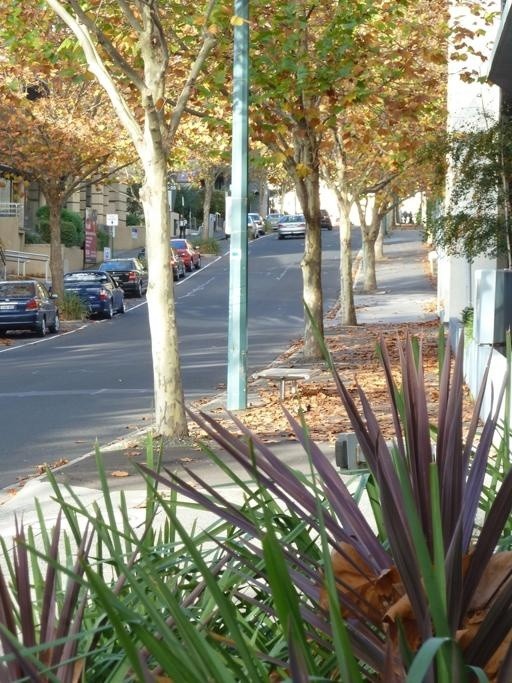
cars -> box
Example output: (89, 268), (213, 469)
(0, 239), (201, 338)
(248, 210), (332, 240)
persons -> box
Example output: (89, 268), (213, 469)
(178, 214), (187, 238)
(403, 211), (412, 223)
